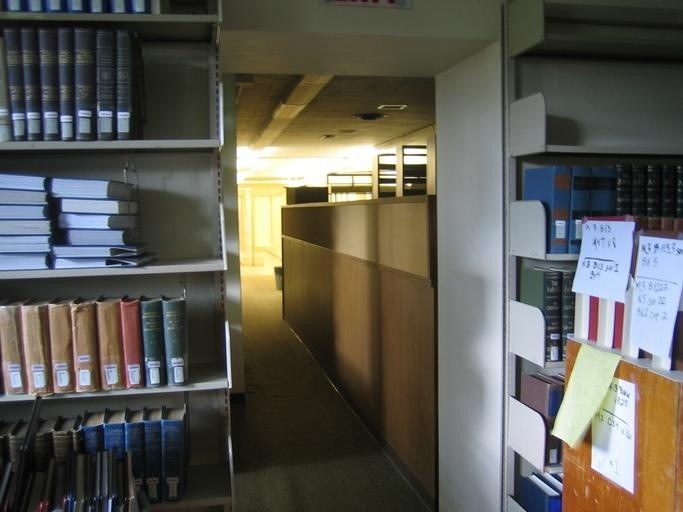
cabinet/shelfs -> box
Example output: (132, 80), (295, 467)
(282, 137), (436, 205)
(559, 335), (682, 512)
(499, 1), (682, 512)
(0, 0), (240, 512)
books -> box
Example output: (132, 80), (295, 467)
(515, 265), (577, 512)
(2, 0), (161, 271)
(0, 293), (193, 511)
(515, 164), (683, 252)
